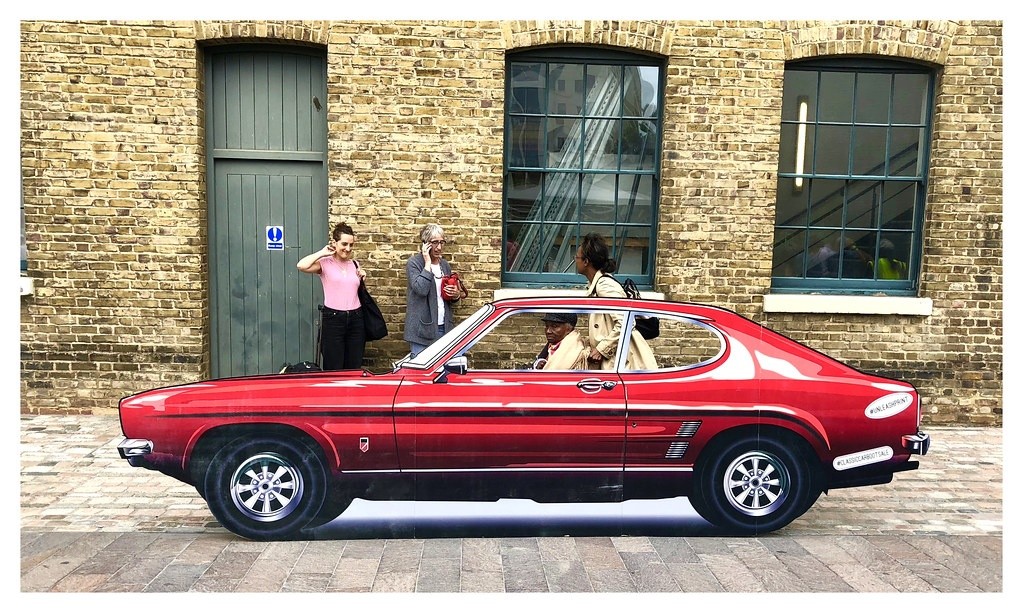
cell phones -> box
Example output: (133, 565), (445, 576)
(422, 240), (431, 254)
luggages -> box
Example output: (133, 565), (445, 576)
(279, 304), (325, 374)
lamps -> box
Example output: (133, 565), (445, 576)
(795, 95), (810, 191)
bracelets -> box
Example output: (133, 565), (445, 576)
(452, 290), (460, 300)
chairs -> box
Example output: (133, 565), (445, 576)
(542, 330), (589, 370)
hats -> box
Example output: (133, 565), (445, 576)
(540, 314), (578, 325)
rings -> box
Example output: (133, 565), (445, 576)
(451, 292), (453, 294)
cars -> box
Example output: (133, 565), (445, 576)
(116, 294), (932, 543)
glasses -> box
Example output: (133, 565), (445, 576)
(429, 240), (446, 245)
(573, 255), (587, 261)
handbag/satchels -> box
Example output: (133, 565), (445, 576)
(441, 272), (468, 301)
(353, 260), (388, 342)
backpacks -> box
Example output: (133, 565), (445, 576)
(623, 279), (660, 340)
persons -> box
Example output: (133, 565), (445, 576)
(402, 222), (468, 360)
(575, 231), (658, 371)
(532, 313), (591, 371)
(297, 221), (366, 371)
(867, 238), (907, 280)
